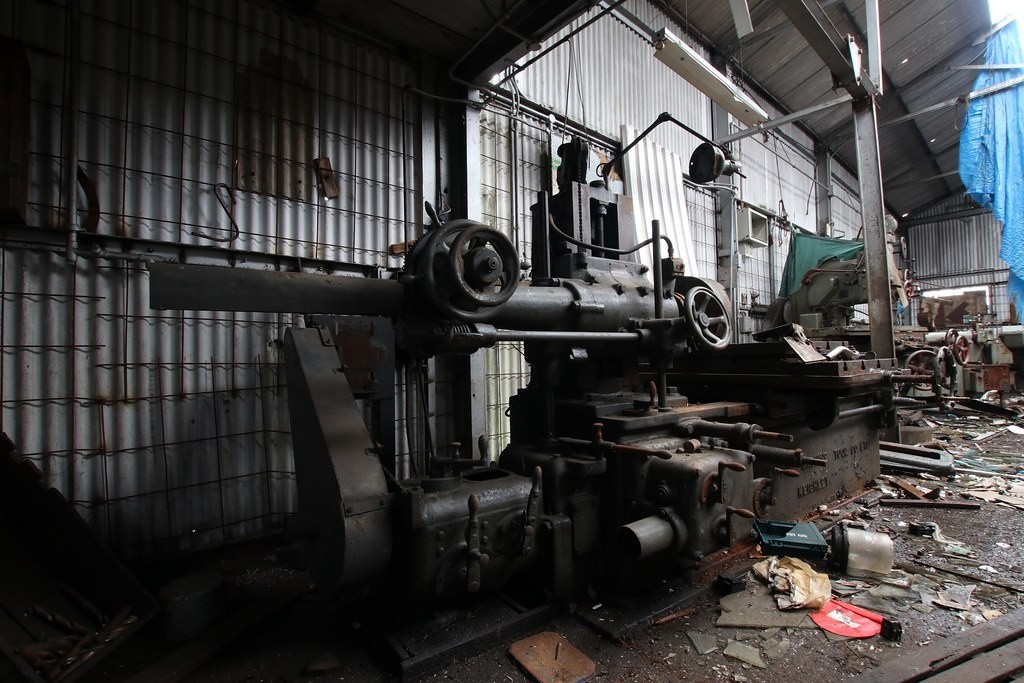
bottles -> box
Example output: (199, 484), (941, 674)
(824, 522), (895, 575)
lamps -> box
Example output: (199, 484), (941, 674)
(649, 26), (769, 130)
(597, 111), (747, 192)
